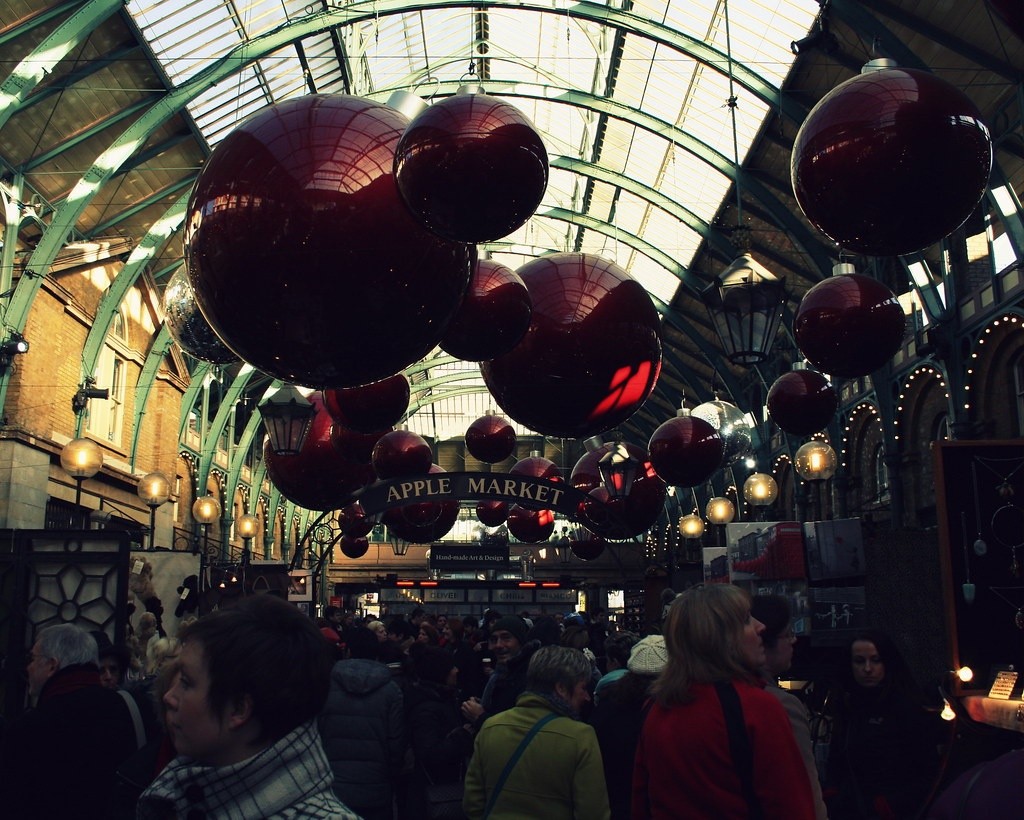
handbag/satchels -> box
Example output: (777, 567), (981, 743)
(425, 782), (464, 820)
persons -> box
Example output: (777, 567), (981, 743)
(738, 596), (829, 820)
(926, 748), (1024, 820)
(821, 628), (937, 820)
(631, 581), (817, 820)
(136, 592), (364, 820)
(0, 612), (196, 820)
(318, 606), (657, 820)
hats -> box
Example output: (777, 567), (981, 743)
(409, 642), (454, 688)
(490, 616), (529, 644)
(89, 631), (127, 684)
(627, 635), (672, 673)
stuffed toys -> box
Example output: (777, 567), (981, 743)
(175, 575), (198, 617)
(126, 555), (167, 638)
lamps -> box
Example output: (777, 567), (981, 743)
(71, 377), (109, 414)
(551, 542), (574, 564)
(0, 328), (29, 376)
(695, 0), (795, 366)
(387, 531), (411, 555)
(257, 380), (321, 457)
(596, 426), (640, 501)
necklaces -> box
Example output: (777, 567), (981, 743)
(974, 456), (1024, 497)
(961, 463), (987, 603)
(990, 587), (1024, 630)
(991, 505), (1024, 576)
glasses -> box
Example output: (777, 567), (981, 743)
(25, 652), (45, 663)
(780, 629), (795, 640)
